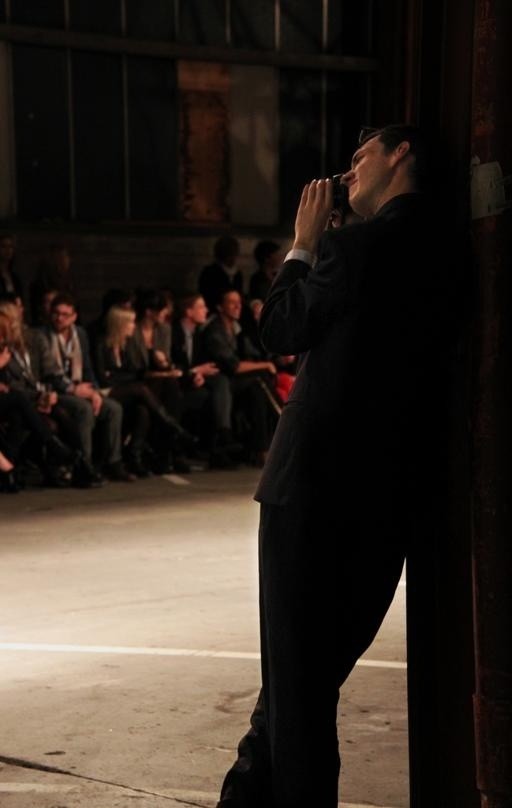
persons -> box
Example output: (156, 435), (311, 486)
(0, 212), (365, 492)
(218, 124), (464, 808)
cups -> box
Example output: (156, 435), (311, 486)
(35, 382), (52, 413)
